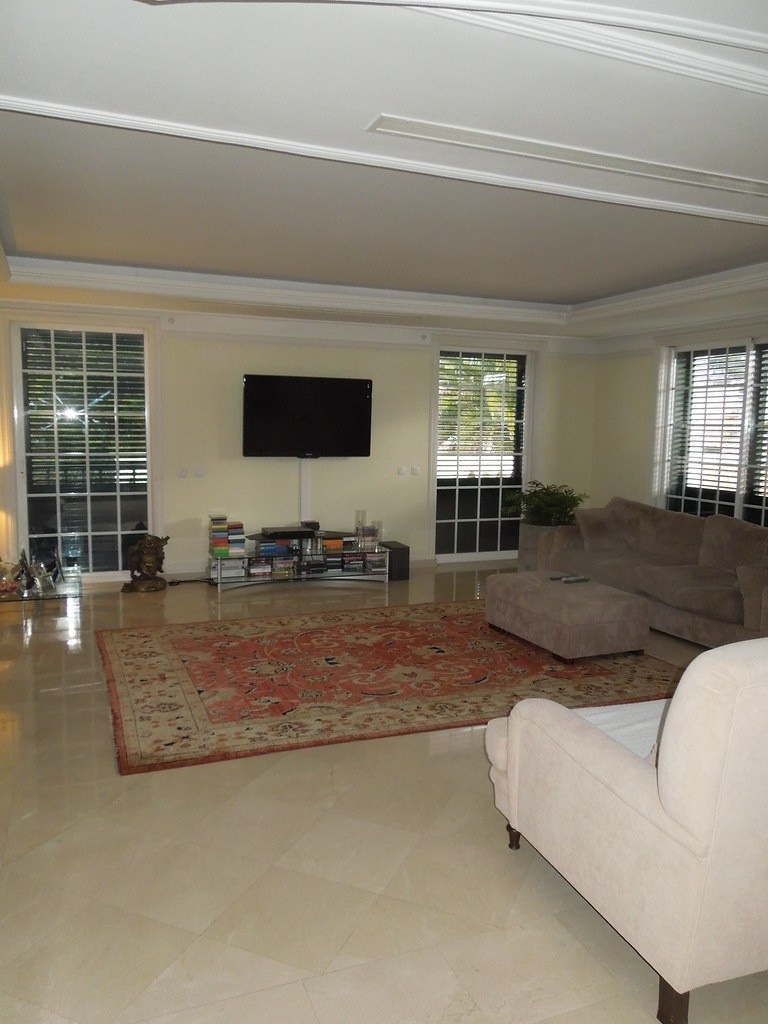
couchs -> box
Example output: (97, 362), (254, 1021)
(543, 497), (768, 650)
(481, 633), (768, 1024)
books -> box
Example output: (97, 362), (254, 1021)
(256, 539), (289, 557)
(205, 558), (387, 576)
(208, 514), (246, 554)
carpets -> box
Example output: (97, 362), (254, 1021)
(91, 598), (693, 778)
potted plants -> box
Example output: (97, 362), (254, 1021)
(514, 481), (583, 562)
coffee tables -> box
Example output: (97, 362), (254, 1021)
(485, 570), (652, 664)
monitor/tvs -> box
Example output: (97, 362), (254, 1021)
(242, 374), (373, 459)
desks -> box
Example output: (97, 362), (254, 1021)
(209, 538), (391, 592)
